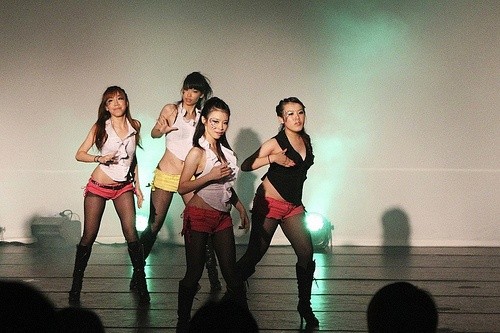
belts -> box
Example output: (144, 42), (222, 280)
(89, 177), (125, 191)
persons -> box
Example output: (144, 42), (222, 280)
(0, 278), (56, 333)
(68, 86), (150, 308)
(176, 300), (260, 333)
(130, 71), (222, 291)
(367, 282), (438, 333)
(175, 96), (249, 333)
(56, 306), (105, 333)
(234, 98), (320, 326)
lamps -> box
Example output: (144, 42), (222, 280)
(302, 211), (336, 255)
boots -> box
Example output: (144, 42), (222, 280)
(68, 244), (92, 301)
(127, 243), (151, 304)
(226, 281), (259, 333)
(296, 261), (319, 326)
(130, 238), (157, 290)
(176, 281), (201, 332)
(236, 254), (256, 281)
(205, 246), (221, 290)
